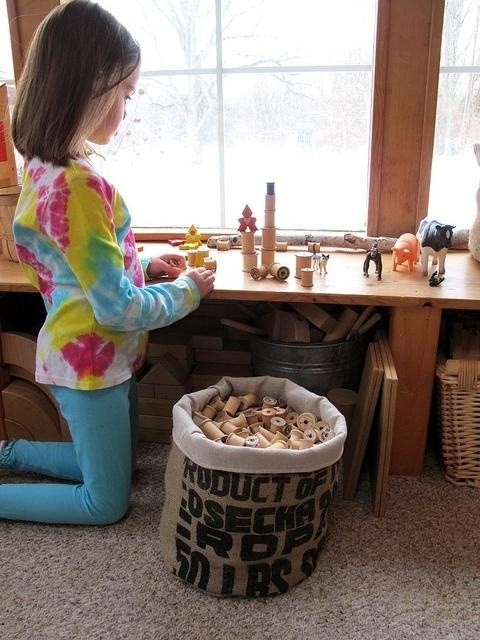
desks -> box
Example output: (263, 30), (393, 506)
(0, 241), (480, 475)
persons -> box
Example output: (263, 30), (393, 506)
(0, 0), (215, 528)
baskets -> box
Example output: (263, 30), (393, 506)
(435, 356), (480, 488)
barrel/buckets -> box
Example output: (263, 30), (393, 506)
(252, 338), (362, 397)
